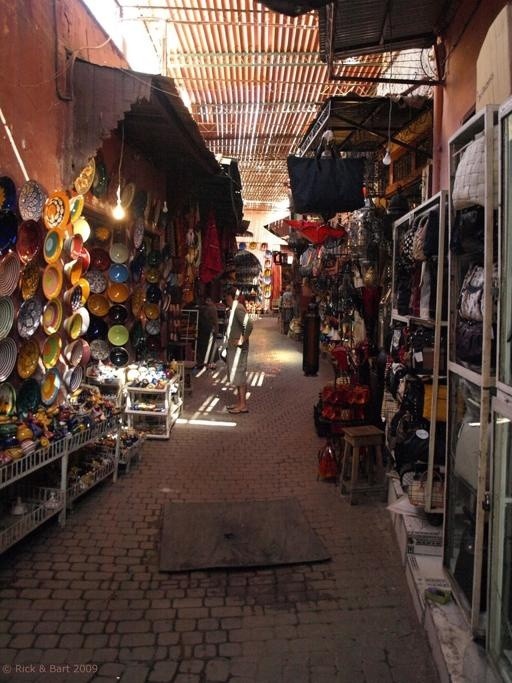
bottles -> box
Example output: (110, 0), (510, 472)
(319, 431), (338, 478)
(171, 358), (177, 368)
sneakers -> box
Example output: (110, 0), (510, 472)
(227, 405), (249, 414)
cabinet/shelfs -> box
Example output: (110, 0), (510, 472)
(1, 362), (185, 553)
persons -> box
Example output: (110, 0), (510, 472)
(279, 285), (293, 311)
(198, 295), (219, 364)
(223, 289), (254, 413)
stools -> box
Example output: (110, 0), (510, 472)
(339, 425), (388, 506)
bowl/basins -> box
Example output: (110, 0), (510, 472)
(0, 150), (173, 462)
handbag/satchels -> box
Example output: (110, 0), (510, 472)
(317, 442), (342, 481)
(286, 137), (365, 215)
(314, 374), (373, 437)
(382, 125), (504, 614)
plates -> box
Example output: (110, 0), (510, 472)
(237, 241), (271, 300)
